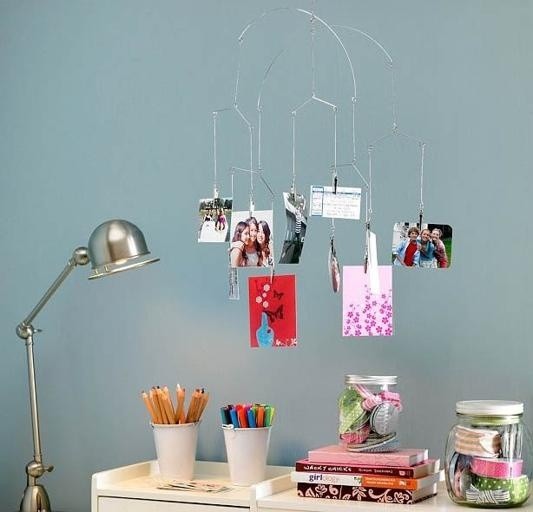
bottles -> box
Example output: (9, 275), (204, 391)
(444, 397), (531, 509)
(337, 374), (409, 455)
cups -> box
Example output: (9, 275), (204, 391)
(145, 418), (209, 484)
(222, 420), (274, 492)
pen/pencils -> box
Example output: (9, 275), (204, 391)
(141, 383), (209, 425)
(220, 403), (274, 428)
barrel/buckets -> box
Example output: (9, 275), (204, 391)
(221, 423), (273, 487)
(149, 419), (203, 483)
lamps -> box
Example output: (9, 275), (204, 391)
(16, 219), (159, 512)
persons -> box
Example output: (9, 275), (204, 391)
(393, 226), (449, 270)
(230, 214), (274, 267)
(284, 201), (306, 258)
(203, 205), (228, 231)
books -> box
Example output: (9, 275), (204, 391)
(290, 445), (440, 504)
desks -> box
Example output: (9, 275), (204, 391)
(91, 459), (533, 512)
